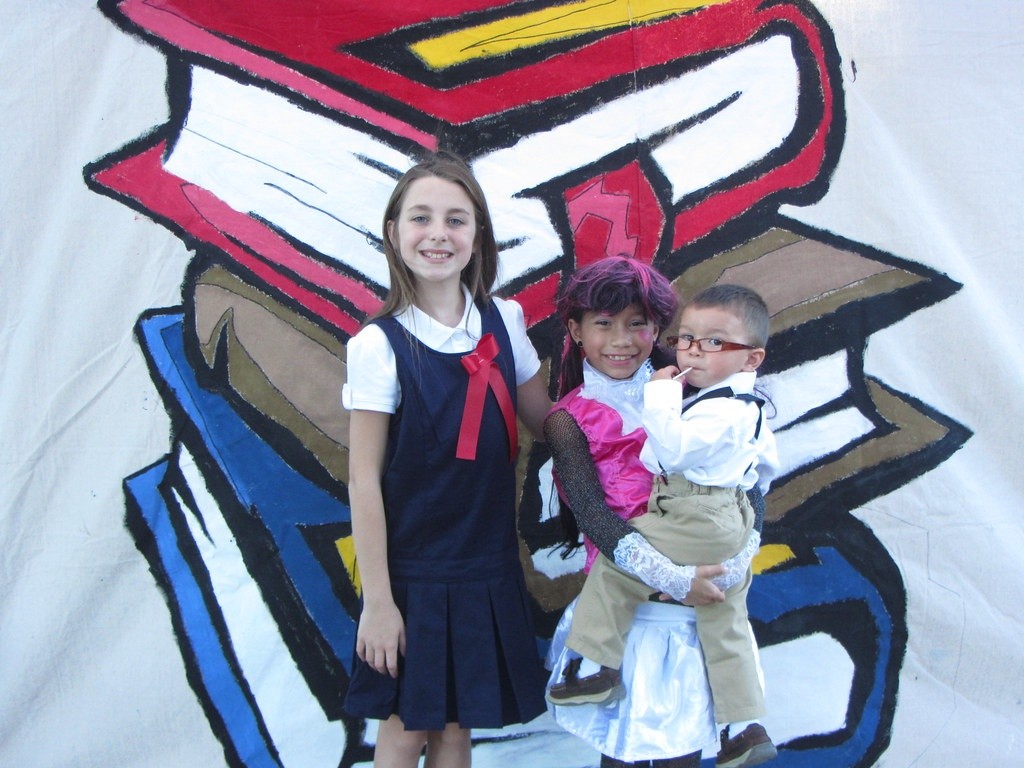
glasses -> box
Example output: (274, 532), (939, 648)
(665, 335), (760, 354)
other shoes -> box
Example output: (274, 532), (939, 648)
(716, 724), (780, 766)
(545, 660), (624, 705)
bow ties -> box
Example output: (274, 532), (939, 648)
(455, 336), (520, 463)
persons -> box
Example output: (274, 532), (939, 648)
(548, 284), (779, 768)
(541, 255), (766, 768)
(341, 159), (559, 768)
